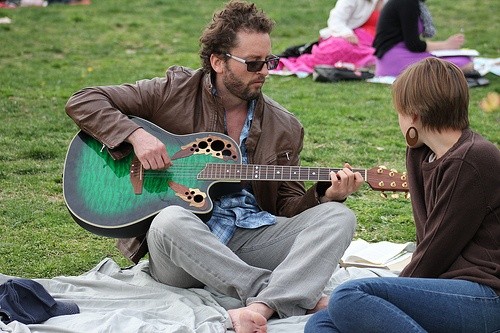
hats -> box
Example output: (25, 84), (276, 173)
(0, 279), (80, 325)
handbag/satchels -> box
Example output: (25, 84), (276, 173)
(313, 63), (363, 82)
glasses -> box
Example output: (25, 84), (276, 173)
(225, 53), (279, 72)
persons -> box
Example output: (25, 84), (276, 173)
(304, 58), (500, 333)
(274, 0), (386, 74)
(372, 0), (475, 79)
(65, 0), (364, 333)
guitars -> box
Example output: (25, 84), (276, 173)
(63, 114), (410, 238)
